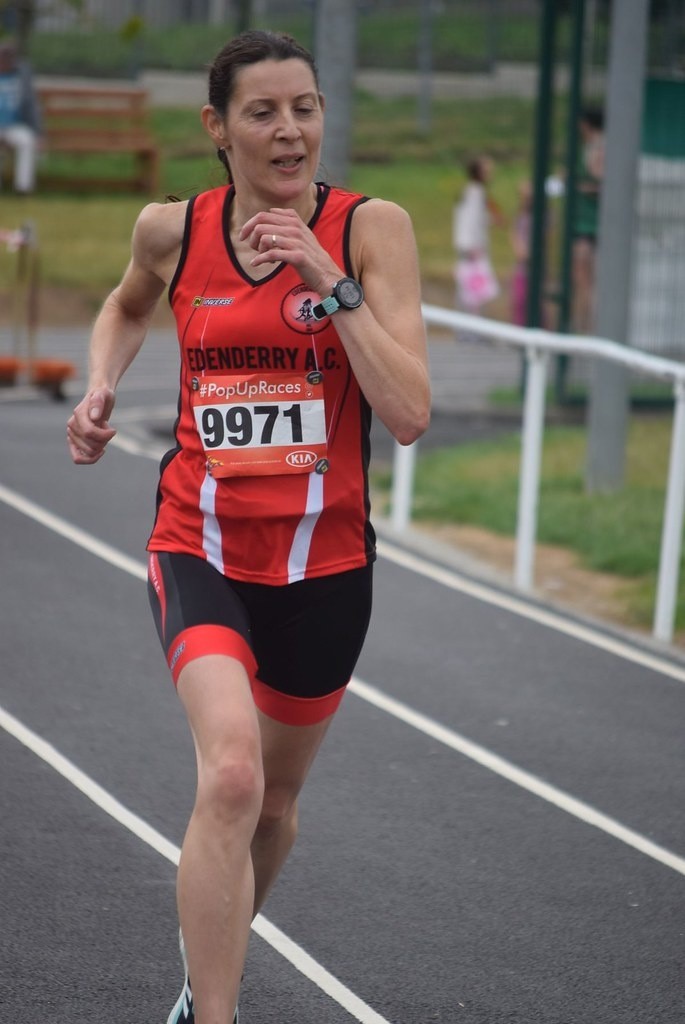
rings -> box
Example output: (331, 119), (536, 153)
(272, 234), (276, 248)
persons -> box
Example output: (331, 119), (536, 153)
(65, 29), (431, 1023)
(451, 103), (607, 331)
(0, 32), (38, 192)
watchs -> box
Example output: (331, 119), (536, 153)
(310, 277), (364, 322)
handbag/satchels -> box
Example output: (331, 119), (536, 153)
(453, 253), (497, 307)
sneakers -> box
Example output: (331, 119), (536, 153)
(167, 923), (240, 1024)
